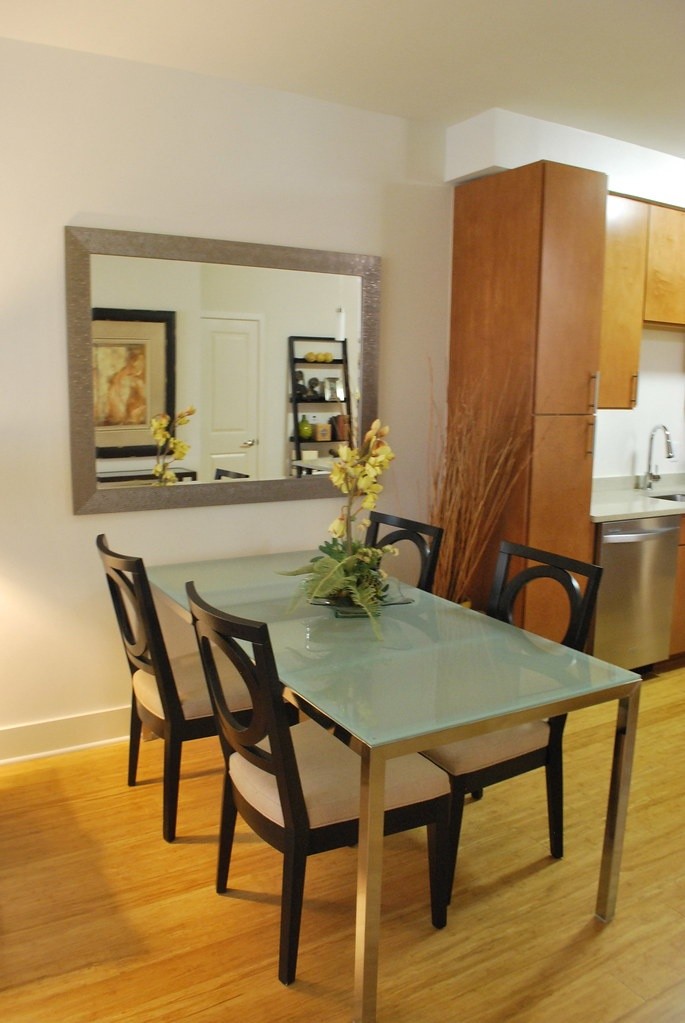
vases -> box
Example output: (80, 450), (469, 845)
(309, 595), (416, 619)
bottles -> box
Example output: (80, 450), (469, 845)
(299, 415), (313, 440)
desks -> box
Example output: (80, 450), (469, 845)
(134, 546), (641, 1023)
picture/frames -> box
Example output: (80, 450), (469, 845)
(89, 307), (176, 459)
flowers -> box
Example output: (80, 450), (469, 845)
(147, 404), (194, 488)
(284, 421), (403, 637)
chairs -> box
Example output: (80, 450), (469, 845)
(359, 511), (445, 592)
(96, 531), (300, 842)
(412, 538), (603, 904)
(184, 579), (452, 986)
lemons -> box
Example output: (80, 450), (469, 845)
(305, 352), (333, 362)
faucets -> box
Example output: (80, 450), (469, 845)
(643, 424), (675, 491)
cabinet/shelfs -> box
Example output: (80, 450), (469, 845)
(286, 332), (354, 475)
(448, 159), (608, 652)
(595, 192), (685, 410)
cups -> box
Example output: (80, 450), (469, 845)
(336, 415), (350, 441)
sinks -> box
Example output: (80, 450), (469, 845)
(648, 493), (685, 503)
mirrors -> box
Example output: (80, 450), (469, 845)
(64, 225), (384, 515)
